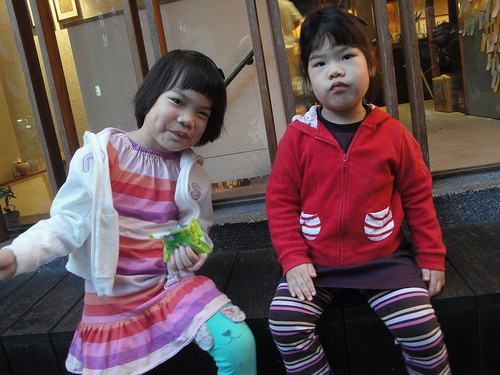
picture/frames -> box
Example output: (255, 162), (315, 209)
(53, 0), (83, 22)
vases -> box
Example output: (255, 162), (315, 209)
(15, 162), (30, 176)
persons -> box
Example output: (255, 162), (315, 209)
(1, 49), (256, 375)
(265, 8), (450, 375)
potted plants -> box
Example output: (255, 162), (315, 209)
(0, 185), (20, 223)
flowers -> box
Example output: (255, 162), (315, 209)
(12, 157), (22, 164)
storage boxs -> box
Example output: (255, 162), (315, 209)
(432, 72), (465, 112)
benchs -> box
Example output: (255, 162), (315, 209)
(0, 221), (500, 375)
